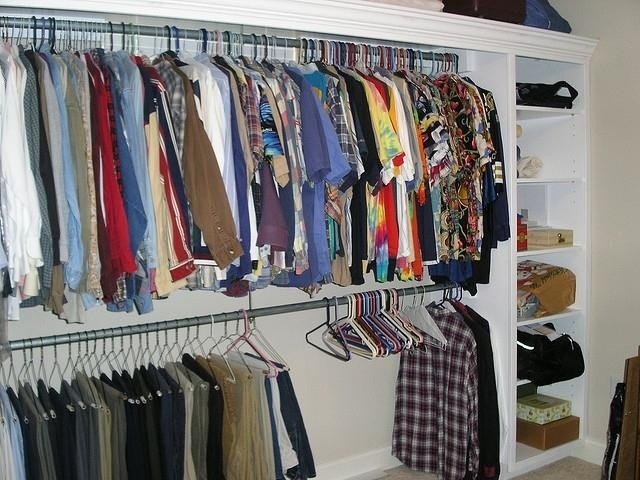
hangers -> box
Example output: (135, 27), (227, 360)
(1, 312), (291, 426)
(304, 276), (485, 361)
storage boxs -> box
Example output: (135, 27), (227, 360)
(516, 376), (581, 452)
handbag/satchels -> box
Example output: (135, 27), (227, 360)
(517, 260), (575, 320)
(517, 323), (584, 385)
(516, 81), (578, 109)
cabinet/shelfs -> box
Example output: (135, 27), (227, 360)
(463, 12), (600, 479)
